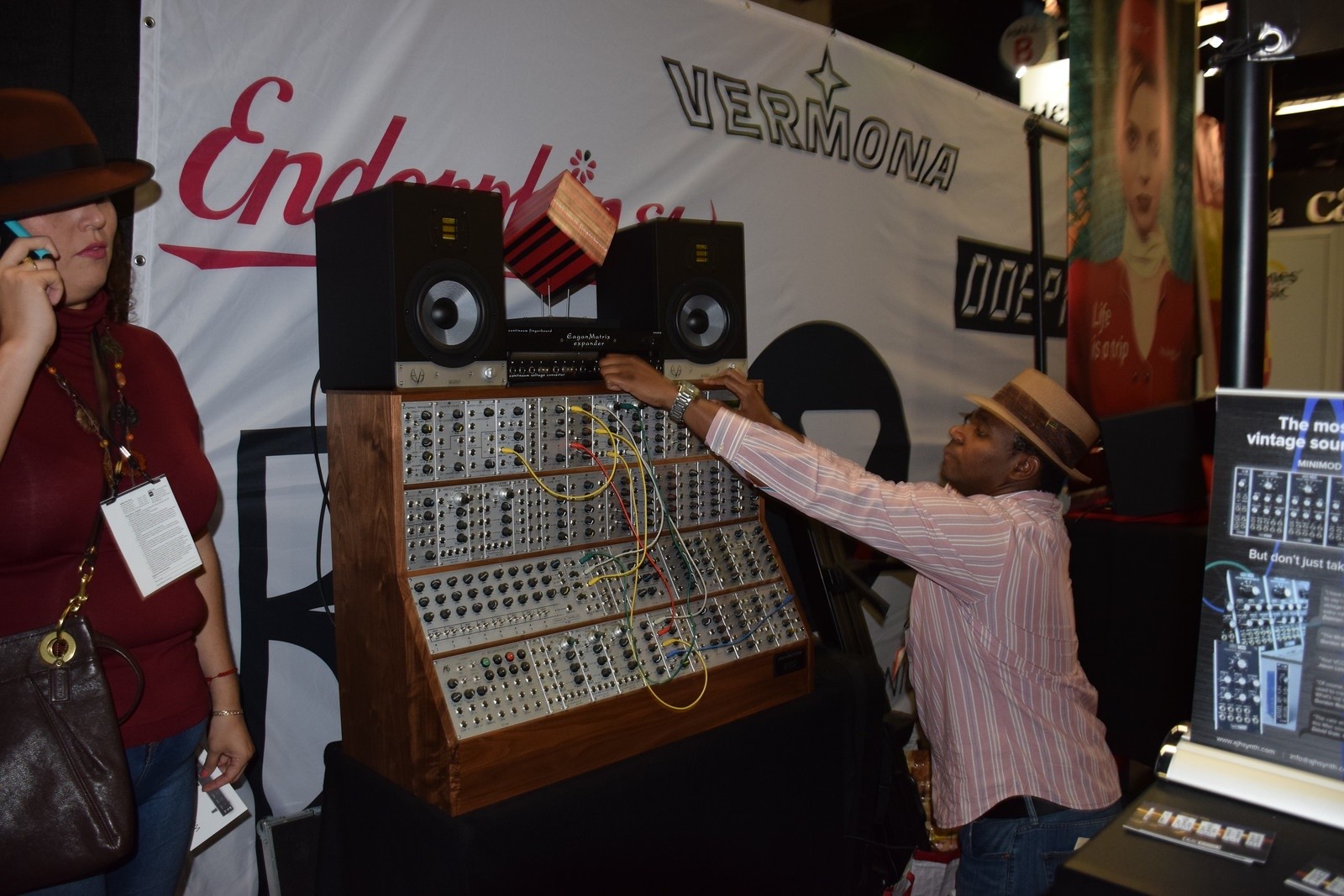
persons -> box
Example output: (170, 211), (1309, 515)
(1066, 0), (1220, 428)
(1, 91), (252, 895)
(597, 354), (1128, 896)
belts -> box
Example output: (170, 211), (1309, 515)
(980, 794), (1069, 821)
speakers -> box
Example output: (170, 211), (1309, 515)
(314, 181), (508, 392)
(596, 217), (749, 382)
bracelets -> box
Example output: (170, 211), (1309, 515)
(203, 667), (238, 689)
(211, 708), (244, 716)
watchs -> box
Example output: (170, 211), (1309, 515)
(667, 380), (702, 426)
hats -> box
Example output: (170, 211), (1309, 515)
(962, 368), (1099, 484)
(0, 87), (156, 223)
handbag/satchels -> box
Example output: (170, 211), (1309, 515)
(0, 617), (139, 896)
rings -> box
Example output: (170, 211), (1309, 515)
(21, 256), (39, 271)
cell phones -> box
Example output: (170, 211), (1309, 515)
(0, 220), (56, 261)
